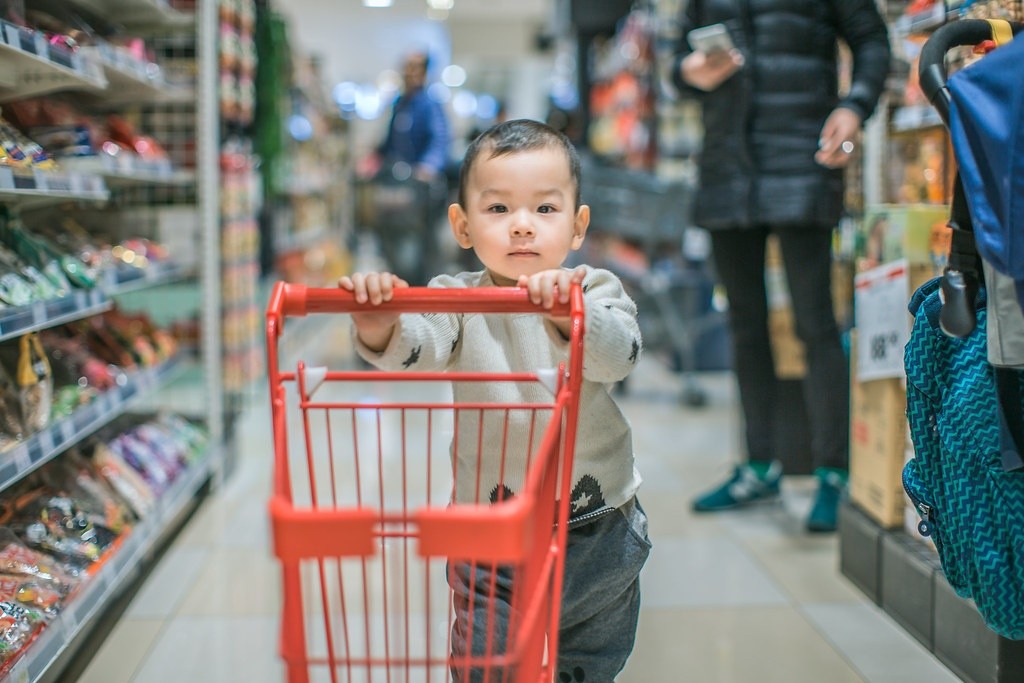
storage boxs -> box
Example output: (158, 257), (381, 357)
(840, 331), (1023, 679)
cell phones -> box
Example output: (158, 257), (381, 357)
(688, 24), (733, 62)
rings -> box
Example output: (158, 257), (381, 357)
(840, 139), (856, 152)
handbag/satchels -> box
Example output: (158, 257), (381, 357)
(896, 220), (1023, 644)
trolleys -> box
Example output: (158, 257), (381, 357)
(353, 156), (709, 410)
(267, 283), (584, 682)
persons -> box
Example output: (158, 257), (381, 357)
(666, 0), (891, 535)
(335, 118), (652, 682)
(359, 45), (455, 248)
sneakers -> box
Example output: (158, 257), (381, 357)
(689, 455), (783, 514)
(805, 466), (847, 536)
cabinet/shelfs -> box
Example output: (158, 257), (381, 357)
(0, 0), (249, 681)
(856, 2), (1023, 269)
